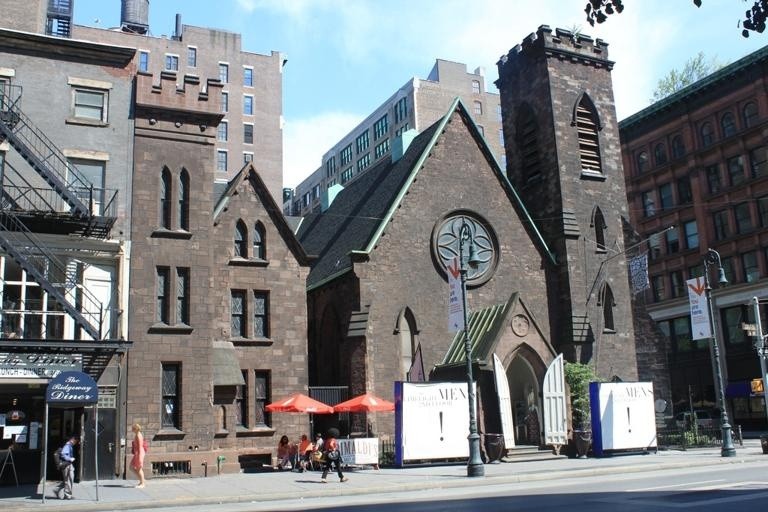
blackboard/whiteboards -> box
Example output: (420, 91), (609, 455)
(0, 450), (9, 477)
(3, 453), (17, 483)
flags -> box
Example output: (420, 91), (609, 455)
(446, 255), (465, 334)
(685, 275), (713, 342)
(626, 249), (652, 296)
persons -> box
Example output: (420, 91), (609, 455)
(277, 433), (325, 470)
(52, 433), (79, 500)
(127, 423), (146, 488)
(320, 427), (348, 482)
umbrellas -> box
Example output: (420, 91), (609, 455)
(264, 391), (334, 461)
(333, 392), (394, 437)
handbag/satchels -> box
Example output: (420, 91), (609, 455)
(324, 450), (342, 462)
(53, 447), (71, 470)
(132, 440), (148, 454)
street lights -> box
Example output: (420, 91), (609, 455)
(458, 221), (484, 478)
(701, 247), (736, 458)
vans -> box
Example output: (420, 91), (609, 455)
(675, 410), (713, 430)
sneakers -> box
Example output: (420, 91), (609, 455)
(321, 479), (327, 483)
(64, 495), (75, 500)
(53, 489), (61, 499)
(340, 478), (348, 482)
(135, 483), (144, 489)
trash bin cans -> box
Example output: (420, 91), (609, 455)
(483, 432), (505, 466)
